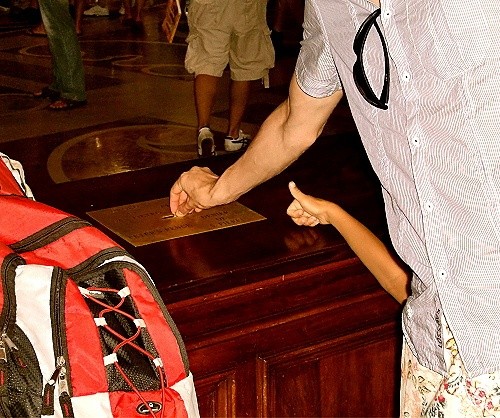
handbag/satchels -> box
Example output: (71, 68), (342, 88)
(0, 154), (201, 418)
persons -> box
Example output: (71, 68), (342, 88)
(124, 0), (144, 24)
(183, 0), (275, 158)
(33, 0), (86, 110)
(286, 181), (412, 304)
(170, 0), (500, 418)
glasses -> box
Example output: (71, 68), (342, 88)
(352, 7), (390, 111)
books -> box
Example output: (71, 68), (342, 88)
(162, 0), (181, 43)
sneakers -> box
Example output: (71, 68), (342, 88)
(198, 126), (216, 156)
(224, 129), (255, 152)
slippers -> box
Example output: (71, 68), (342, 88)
(49, 96), (88, 111)
(33, 86), (62, 100)
